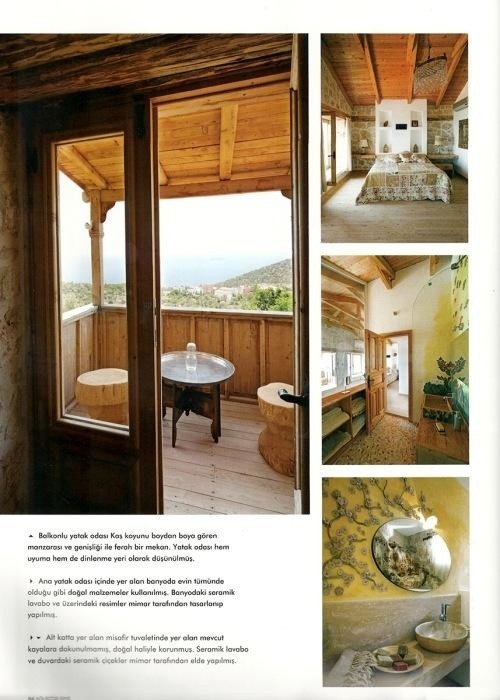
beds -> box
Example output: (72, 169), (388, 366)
(357, 152), (452, 204)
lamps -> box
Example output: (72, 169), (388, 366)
(413, 44), (447, 96)
(359, 139), (368, 155)
(433, 136), (444, 154)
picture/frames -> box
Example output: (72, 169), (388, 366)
(459, 120), (468, 149)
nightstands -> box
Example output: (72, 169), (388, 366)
(427, 153), (458, 177)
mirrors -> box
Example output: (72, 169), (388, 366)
(371, 520), (453, 593)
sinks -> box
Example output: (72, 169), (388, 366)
(415, 621), (468, 653)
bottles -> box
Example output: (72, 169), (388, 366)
(185, 343), (198, 373)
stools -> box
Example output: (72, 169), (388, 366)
(75, 366), (130, 425)
(255, 382), (295, 478)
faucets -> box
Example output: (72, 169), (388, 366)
(439, 603), (451, 621)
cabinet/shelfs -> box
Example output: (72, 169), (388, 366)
(379, 111), (393, 153)
(410, 110), (423, 153)
(321, 384), (365, 466)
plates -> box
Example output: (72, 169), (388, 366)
(372, 644), (425, 675)
